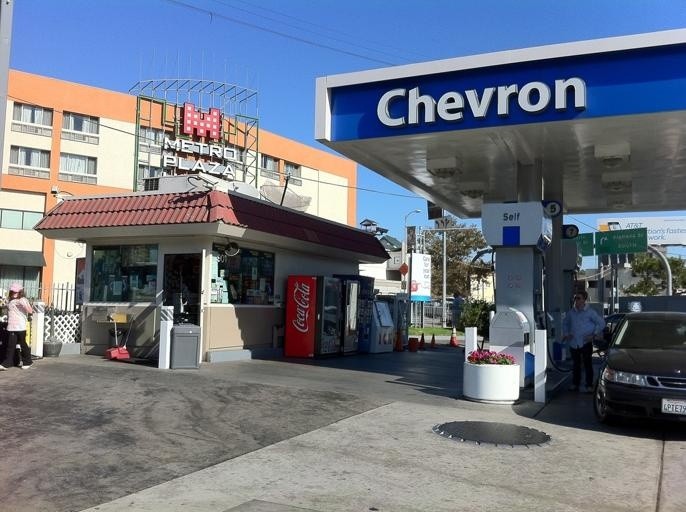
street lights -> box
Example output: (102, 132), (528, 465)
(401, 207), (421, 264)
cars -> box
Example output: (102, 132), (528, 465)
(591, 311), (686, 424)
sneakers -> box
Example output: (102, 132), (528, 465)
(0, 365), (9, 371)
(21, 364), (32, 369)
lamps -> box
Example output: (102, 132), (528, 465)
(426, 157), (463, 180)
(592, 139), (632, 171)
(458, 180), (490, 199)
(601, 172), (632, 192)
(607, 191), (637, 209)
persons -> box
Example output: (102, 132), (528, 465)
(450, 290), (465, 326)
(0, 282), (35, 372)
(560, 290), (606, 392)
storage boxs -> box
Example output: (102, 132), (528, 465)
(109, 312), (133, 323)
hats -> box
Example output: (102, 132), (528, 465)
(10, 283), (22, 293)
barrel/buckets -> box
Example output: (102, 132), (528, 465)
(408, 338), (418, 353)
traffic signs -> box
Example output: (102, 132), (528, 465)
(594, 227), (648, 256)
(576, 231), (594, 257)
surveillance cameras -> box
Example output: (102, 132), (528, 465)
(51, 186), (59, 194)
(197, 171), (219, 186)
(376, 227), (388, 233)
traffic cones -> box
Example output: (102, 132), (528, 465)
(429, 334), (439, 348)
(446, 325), (462, 348)
(393, 335), (404, 351)
(419, 332), (426, 349)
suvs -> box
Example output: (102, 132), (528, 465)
(432, 297), (454, 307)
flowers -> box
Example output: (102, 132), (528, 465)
(467, 349), (517, 365)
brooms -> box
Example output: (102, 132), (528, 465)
(117, 314), (135, 359)
